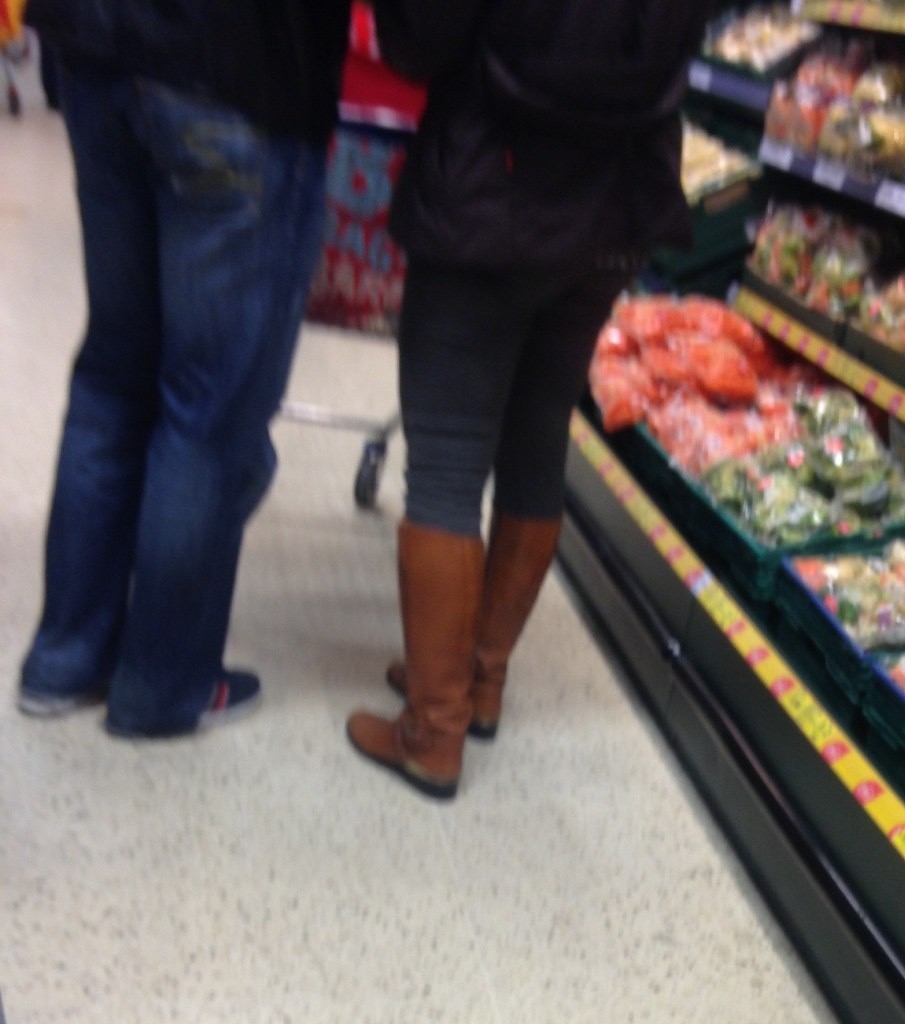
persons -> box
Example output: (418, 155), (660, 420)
(17, 1), (354, 741)
(345, 1), (717, 799)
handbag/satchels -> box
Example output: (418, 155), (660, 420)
(291, 1), (426, 340)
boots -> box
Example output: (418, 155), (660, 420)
(387, 506), (563, 736)
(347, 521), (485, 794)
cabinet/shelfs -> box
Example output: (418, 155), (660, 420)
(550, 0), (905, 1024)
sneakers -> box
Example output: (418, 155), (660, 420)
(18, 681), (109, 715)
(105, 664), (262, 740)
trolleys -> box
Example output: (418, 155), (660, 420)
(0, 1), (33, 117)
(277, 1), (429, 512)
